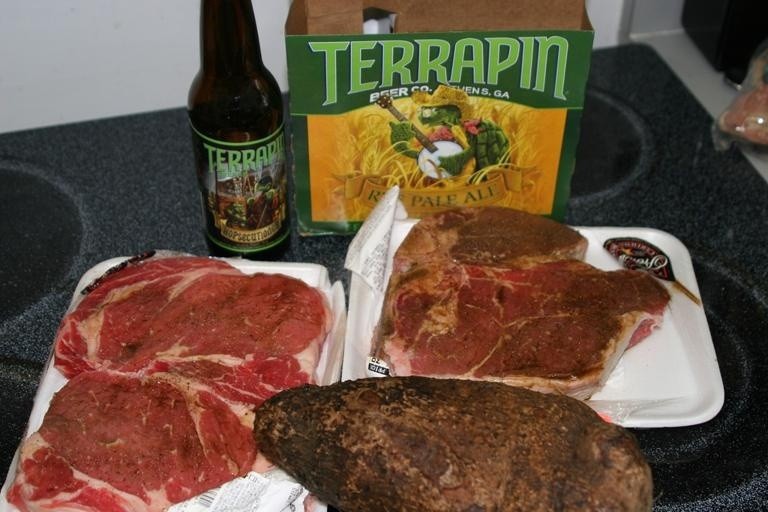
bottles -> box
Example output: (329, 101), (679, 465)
(186, 0), (291, 258)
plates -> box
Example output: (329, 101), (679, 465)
(0, 256), (346, 512)
(340, 220), (724, 429)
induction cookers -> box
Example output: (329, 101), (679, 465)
(0, 40), (767, 512)
